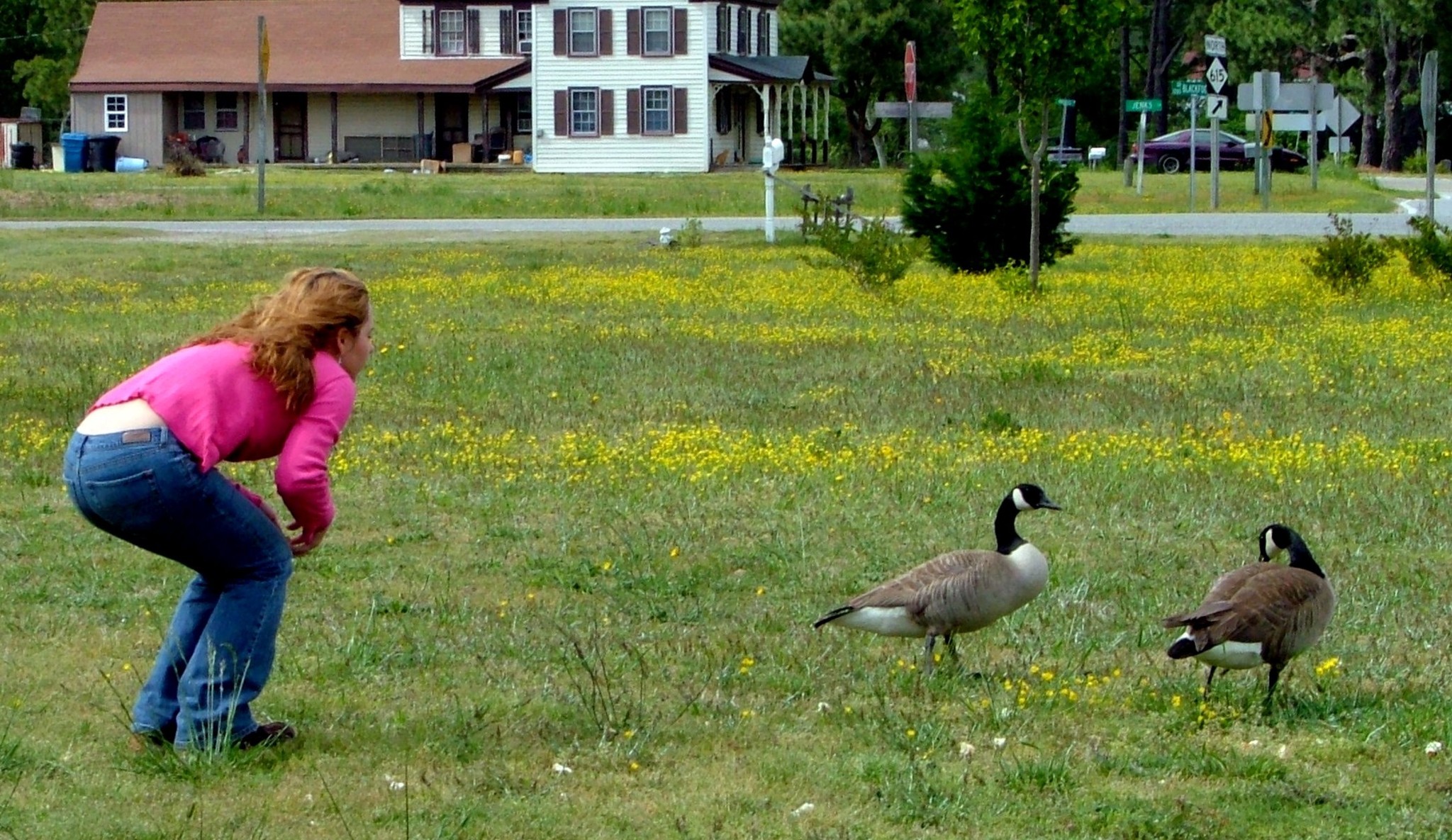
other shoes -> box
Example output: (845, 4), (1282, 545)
(125, 732), (174, 757)
(216, 722), (295, 754)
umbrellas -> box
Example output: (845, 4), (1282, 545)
(904, 42), (916, 169)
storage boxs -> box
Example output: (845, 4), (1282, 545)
(512, 148), (524, 163)
(498, 154), (511, 163)
(452, 143), (475, 163)
(421, 159), (447, 174)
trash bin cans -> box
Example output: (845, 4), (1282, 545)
(52, 143), (63, 171)
(115, 156), (148, 172)
(60, 133), (86, 173)
(87, 135), (122, 172)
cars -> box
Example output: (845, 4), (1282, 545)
(1130, 128), (1308, 175)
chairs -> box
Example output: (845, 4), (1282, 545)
(488, 126), (507, 159)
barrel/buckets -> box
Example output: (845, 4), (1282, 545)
(498, 154), (511, 165)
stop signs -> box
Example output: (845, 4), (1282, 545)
(904, 40), (918, 103)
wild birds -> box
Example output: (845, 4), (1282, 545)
(812, 482), (1063, 672)
(1168, 524), (1336, 714)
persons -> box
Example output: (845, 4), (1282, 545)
(61, 268), (377, 757)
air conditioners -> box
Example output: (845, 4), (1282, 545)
(519, 40), (532, 54)
